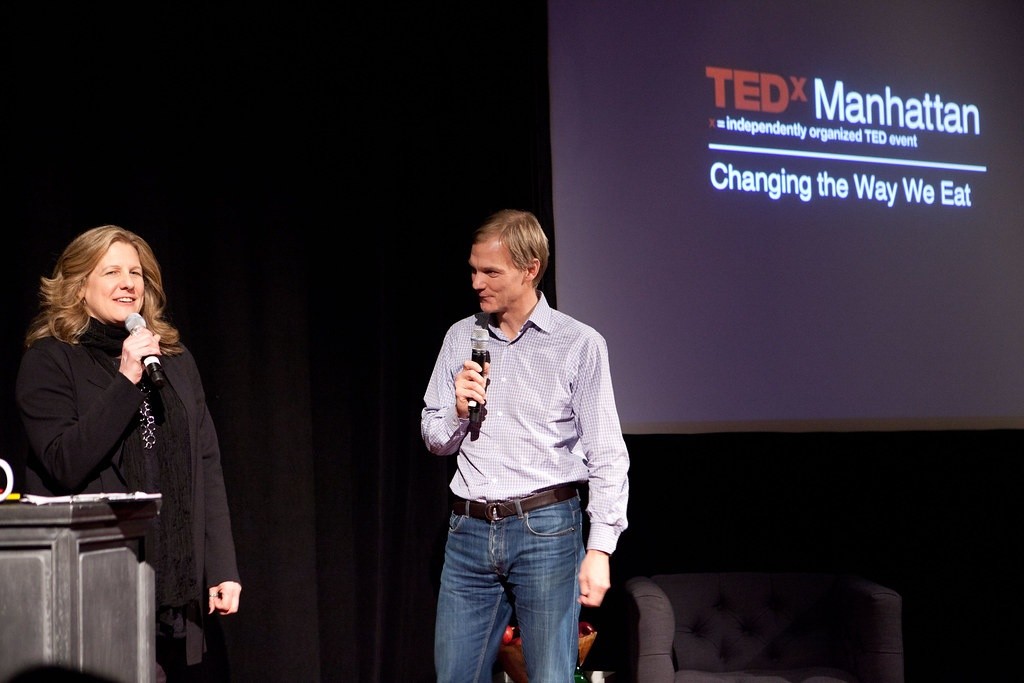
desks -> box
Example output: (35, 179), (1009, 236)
(0, 500), (163, 683)
(583, 671), (615, 683)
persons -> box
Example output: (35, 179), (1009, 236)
(421, 210), (629, 683)
(15, 226), (243, 682)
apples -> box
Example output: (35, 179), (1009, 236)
(503, 622), (594, 645)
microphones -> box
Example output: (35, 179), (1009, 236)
(125, 313), (163, 383)
(469, 329), (489, 422)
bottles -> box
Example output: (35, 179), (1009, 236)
(574, 649), (588, 683)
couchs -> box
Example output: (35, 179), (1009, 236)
(619, 572), (905, 683)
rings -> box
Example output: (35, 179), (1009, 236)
(130, 330), (138, 337)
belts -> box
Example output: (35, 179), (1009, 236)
(454, 485), (577, 521)
